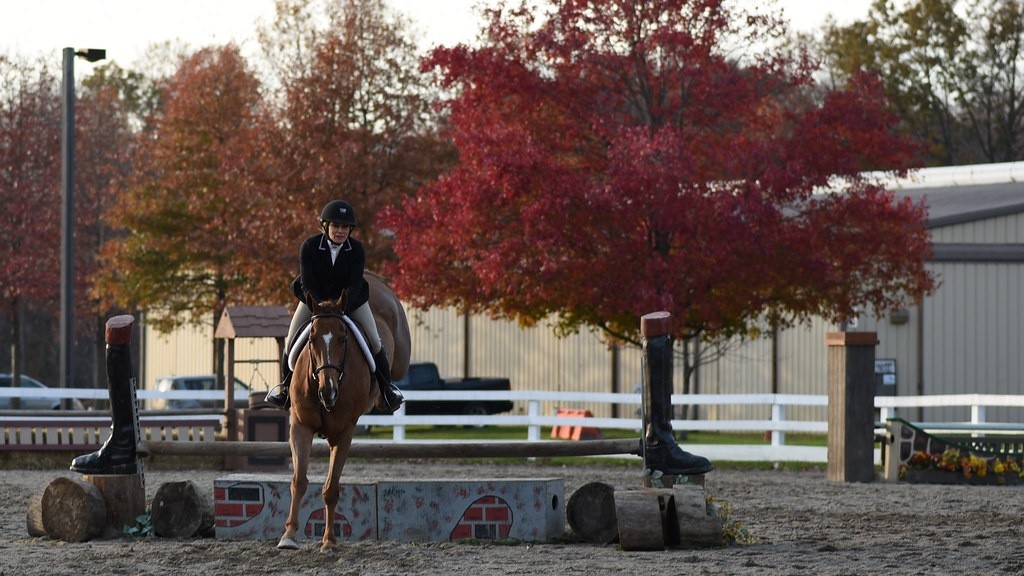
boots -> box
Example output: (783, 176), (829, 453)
(70, 343), (141, 474)
(268, 353), (292, 411)
(643, 332), (713, 475)
(373, 345), (403, 409)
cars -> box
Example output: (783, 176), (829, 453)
(0, 373), (86, 412)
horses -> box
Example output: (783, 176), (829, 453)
(277, 270), (411, 556)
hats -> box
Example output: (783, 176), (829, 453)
(320, 200), (358, 226)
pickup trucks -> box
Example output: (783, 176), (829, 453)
(361, 361), (515, 433)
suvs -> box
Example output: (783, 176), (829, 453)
(147, 374), (252, 413)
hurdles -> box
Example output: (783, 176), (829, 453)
(129, 369), (644, 459)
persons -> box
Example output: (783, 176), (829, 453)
(265, 199), (404, 409)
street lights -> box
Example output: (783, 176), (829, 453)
(61, 47), (106, 411)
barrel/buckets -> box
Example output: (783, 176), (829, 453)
(248, 369), (269, 408)
